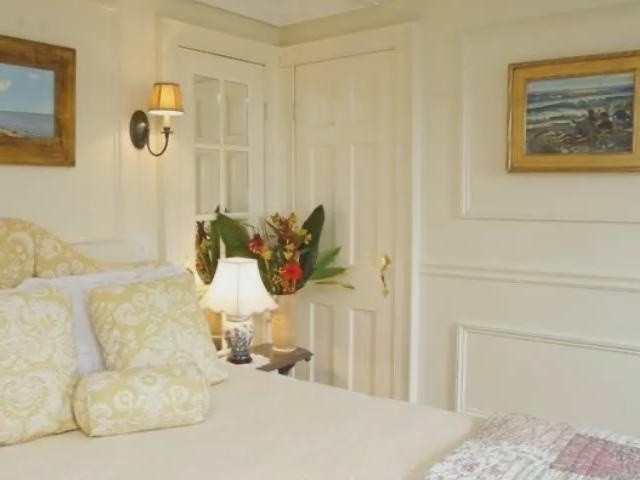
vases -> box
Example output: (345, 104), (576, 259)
(269, 294), (298, 352)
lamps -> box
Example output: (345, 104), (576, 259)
(199, 254), (279, 364)
(129, 82), (184, 157)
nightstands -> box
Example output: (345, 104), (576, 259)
(219, 342), (313, 378)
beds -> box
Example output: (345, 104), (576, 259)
(0, 217), (640, 480)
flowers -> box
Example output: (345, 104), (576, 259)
(192, 199), (355, 299)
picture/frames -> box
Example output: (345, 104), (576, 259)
(0, 34), (77, 168)
(505, 49), (640, 174)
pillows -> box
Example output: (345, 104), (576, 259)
(0, 287), (80, 446)
(87, 273), (228, 386)
(73, 361), (211, 436)
